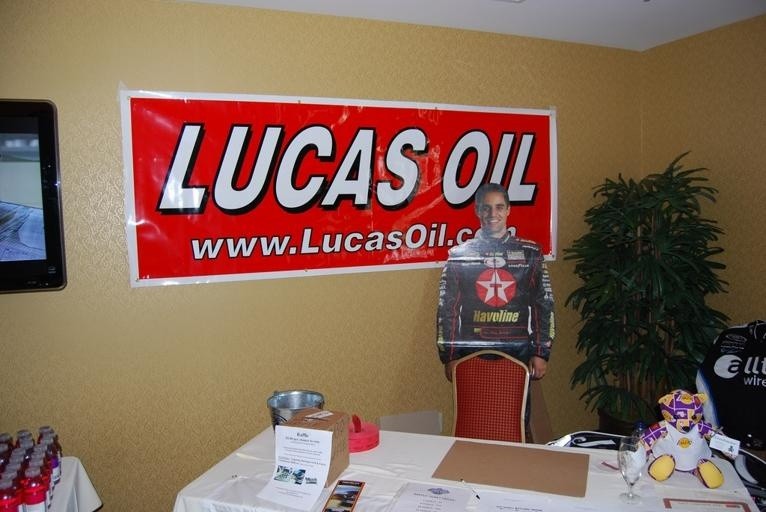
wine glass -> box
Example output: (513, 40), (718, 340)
(618, 436), (646, 505)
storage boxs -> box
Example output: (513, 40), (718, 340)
(286, 406), (349, 487)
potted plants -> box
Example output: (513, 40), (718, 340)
(563, 150), (734, 449)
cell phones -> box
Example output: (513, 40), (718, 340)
(322, 480), (365, 511)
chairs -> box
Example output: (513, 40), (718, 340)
(445, 351), (528, 447)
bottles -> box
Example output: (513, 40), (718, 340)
(632, 425), (650, 462)
(1, 424), (63, 511)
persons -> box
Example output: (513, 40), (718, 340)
(434, 182), (553, 441)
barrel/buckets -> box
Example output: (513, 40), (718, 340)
(266, 389), (325, 433)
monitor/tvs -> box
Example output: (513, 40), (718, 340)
(1, 98), (68, 294)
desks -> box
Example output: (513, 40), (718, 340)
(172, 420), (759, 512)
(1, 455), (102, 512)
